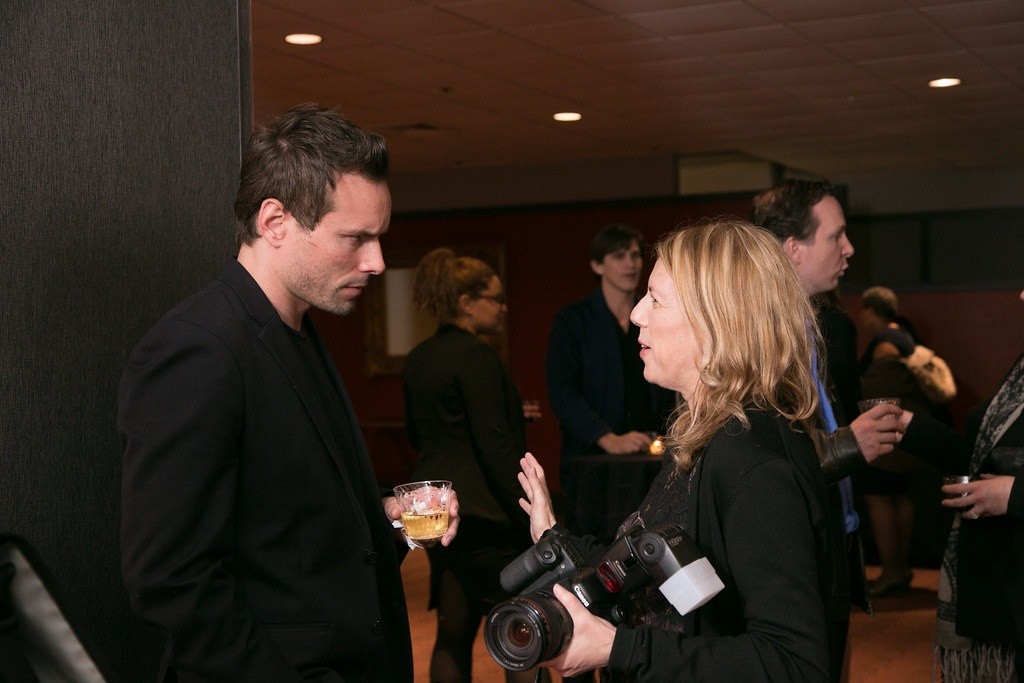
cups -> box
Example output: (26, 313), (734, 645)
(392, 481), (452, 540)
(943, 474), (977, 513)
(860, 399), (903, 432)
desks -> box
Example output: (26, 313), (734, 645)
(359, 421), (413, 469)
(577, 455), (664, 532)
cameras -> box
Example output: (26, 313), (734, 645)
(484, 520), (722, 672)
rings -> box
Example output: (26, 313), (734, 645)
(970, 507), (981, 520)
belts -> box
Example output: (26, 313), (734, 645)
(846, 531), (860, 554)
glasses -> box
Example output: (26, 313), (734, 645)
(467, 293), (504, 305)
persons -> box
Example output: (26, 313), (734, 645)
(117, 101), (460, 683)
(518, 216), (856, 683)
(543, 177), (1023, 682)
(401, 248), (554, 683)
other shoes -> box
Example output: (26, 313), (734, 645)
(868, 570), (914, 598)
